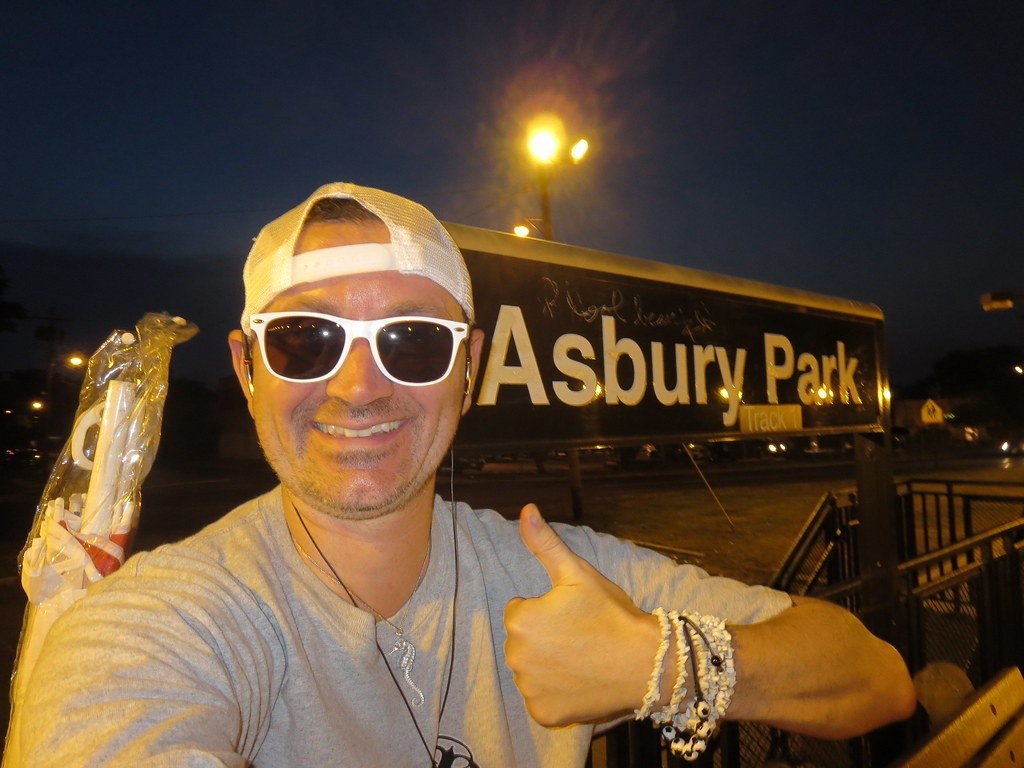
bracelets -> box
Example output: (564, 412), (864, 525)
(635, 606), (737, 760)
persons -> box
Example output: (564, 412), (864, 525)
(18, 181), (916, 768)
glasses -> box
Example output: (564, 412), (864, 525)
(250, 311), (470, 386)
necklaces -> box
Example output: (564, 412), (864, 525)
(289, 526), (431, 707)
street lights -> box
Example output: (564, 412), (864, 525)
(516, 119), (588, 244)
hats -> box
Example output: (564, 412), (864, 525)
(240, 182), (475, 335)
